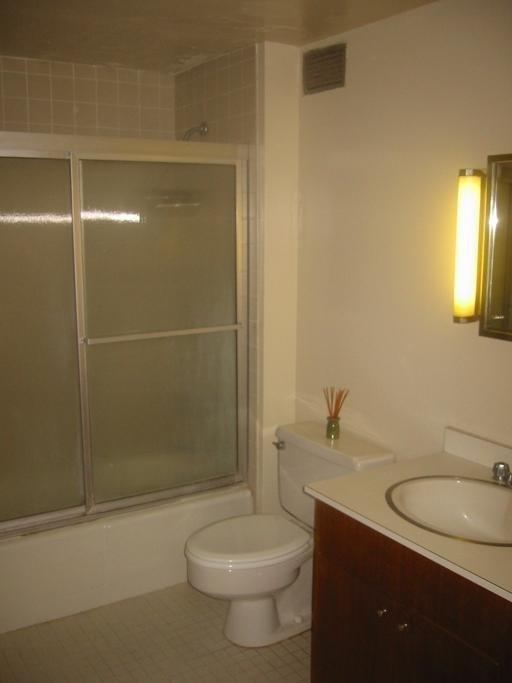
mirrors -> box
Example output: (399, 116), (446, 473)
(479, 154), (512, 344)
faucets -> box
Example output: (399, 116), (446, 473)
(497, 470), (512, 489)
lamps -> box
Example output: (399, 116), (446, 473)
(452, 169), (488, 324)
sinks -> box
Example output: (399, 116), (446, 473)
(385, 474), (512, 548)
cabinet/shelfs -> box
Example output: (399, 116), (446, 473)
(310, 500), (512, 683)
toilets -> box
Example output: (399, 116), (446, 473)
(184, 419), (396, 649)
